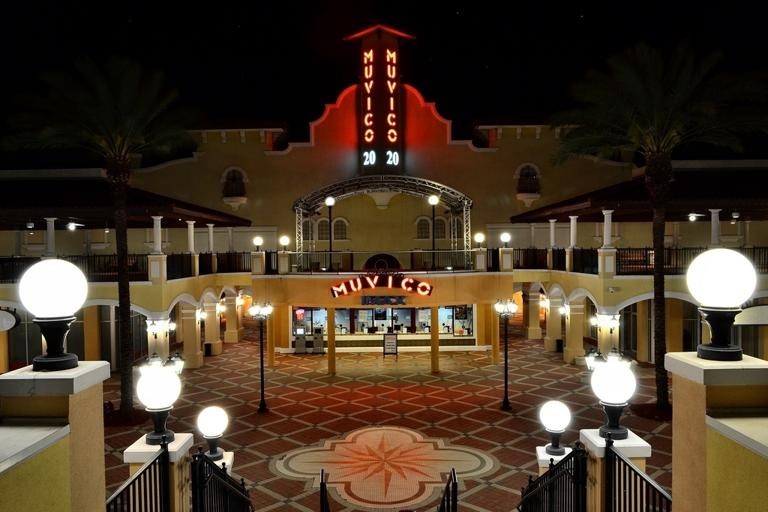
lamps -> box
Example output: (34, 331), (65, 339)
(146, 295), (244, 339)
(538, 297), (618, 334)
(687, 210), (740, 224)
(26, 216), (85, 235)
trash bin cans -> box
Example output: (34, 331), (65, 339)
(556, 339), (563, 352)
(204, 343), (211, 357)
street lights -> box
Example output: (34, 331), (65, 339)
(492, 296), (520, 413)
(427, 194), (440, 270)
(246, 300), (275, 415)
(324, 194), (338, 273)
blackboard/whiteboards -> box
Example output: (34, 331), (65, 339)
(384, 334), (397, 355)
